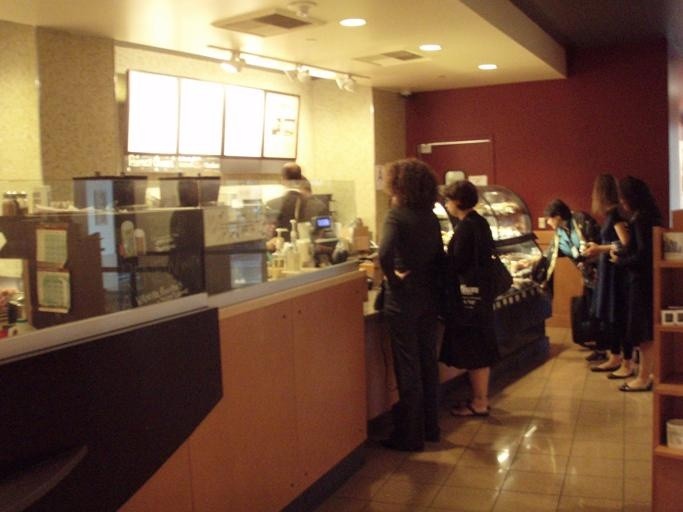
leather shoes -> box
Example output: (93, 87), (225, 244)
(382, 433), (422, 450)
(423, 424), (441, 442)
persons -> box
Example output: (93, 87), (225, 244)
(580, 174), (634, 379)
(266, 162), (311, 242)
(379, 160), (443, 451)
(438, 181), (501, 417)
(609, 176), (660, 392)
(517, 199), (609, 361)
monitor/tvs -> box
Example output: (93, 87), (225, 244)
(295, 195), (331, 224)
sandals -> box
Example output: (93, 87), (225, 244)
(609, 371), (637, 379)
(619, 380), (654, 393)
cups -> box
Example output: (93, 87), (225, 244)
(298, 241), (315, 264)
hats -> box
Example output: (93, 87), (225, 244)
(592, 360), (615, 370)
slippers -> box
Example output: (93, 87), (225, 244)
(455, 399), (471, 407)
(451, 405), (490, 416)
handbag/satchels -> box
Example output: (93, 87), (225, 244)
(491, 250), (513, 295)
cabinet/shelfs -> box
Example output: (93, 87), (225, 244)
(433, 184), (553, 383)
(652, 210), (683, 511)
